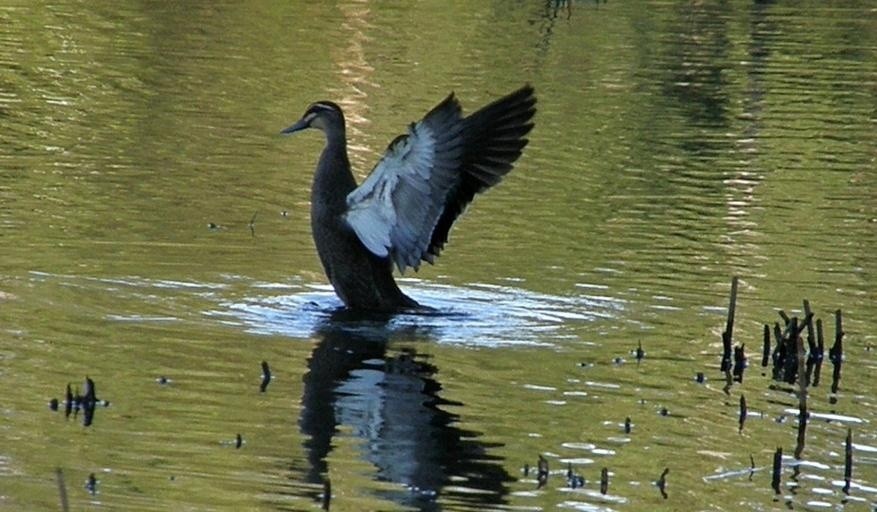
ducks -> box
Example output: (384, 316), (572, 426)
(291, 313), (518, 511)
(282, 84), (558, 312)
